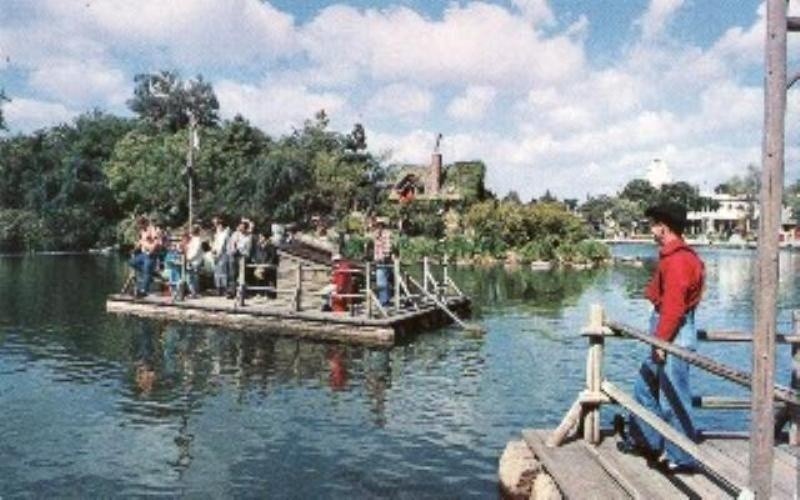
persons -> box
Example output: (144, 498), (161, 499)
(618, 202), (703, 475)
(125, 315), (395, 425)
(125, 206), (399, 313)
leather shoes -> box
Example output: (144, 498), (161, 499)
(617, 441), (649, 454)
(658, 460), (695, 473)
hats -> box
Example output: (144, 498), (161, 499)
(646, 204), (686, 222)
